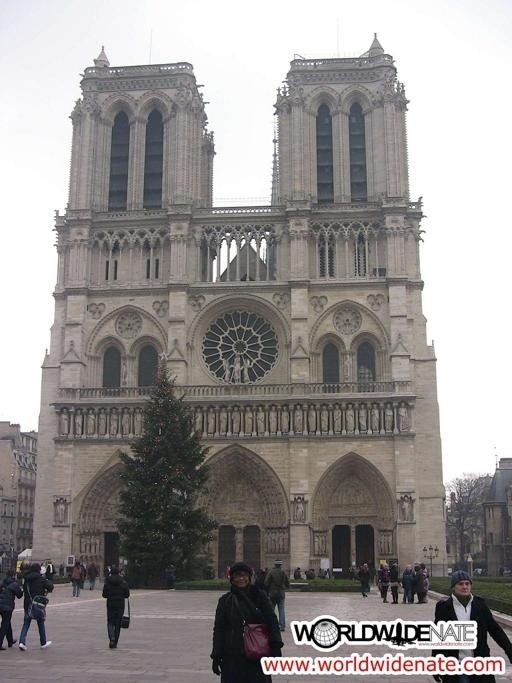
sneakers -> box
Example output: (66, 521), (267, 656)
(9, 640), (17, 647)
(0, 647), (7, 650)
(41, 640), (52, 648)
(279, 625), (285, 631)
(19, 642), (28, 651)
(109, 640), (118, 649)
(382, 600), (428, 604)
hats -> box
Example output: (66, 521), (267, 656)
(274, 559), (283, 565)
(229, 562), (253, 576)
(451, 570), (472, 589)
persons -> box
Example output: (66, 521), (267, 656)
(210, 559), (331, 683)
(348, 560), (430, 604)
(473, 566), (505, 577)
(430, 570), (512, 683)
(0, 560), (129, 651)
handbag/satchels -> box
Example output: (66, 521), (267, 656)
(120, 616), (130, 628)
(28, 594), (50, 620)
(242, 622), (272, 661)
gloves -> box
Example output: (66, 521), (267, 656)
(212, 656), (224, 676)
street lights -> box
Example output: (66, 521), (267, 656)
(423, 544), (439, 576)
